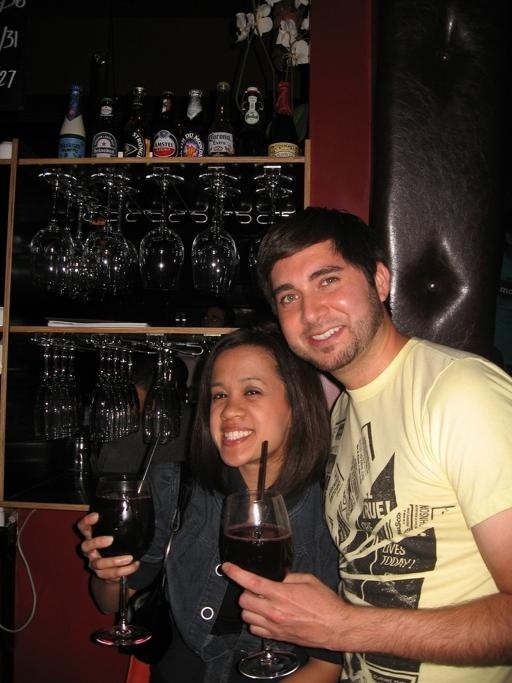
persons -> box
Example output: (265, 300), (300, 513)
(89, 349), (185, 477)
(195, 304), (234, 330)
(218, 203), (512, 683)
(76, 321), (348, 681)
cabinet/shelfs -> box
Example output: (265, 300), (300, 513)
(3, 138), (313, 511)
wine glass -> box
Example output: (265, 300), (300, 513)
(71, 435), (91, 480)
(32, 334), (183, 443)
(88, 476), (156, 650)
(30, 166), (285, 296)
(220, 492), (303, 680)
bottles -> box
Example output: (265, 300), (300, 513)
(58, 85), (298, 159)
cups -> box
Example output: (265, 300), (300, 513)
(0, 140), (15, 158)
